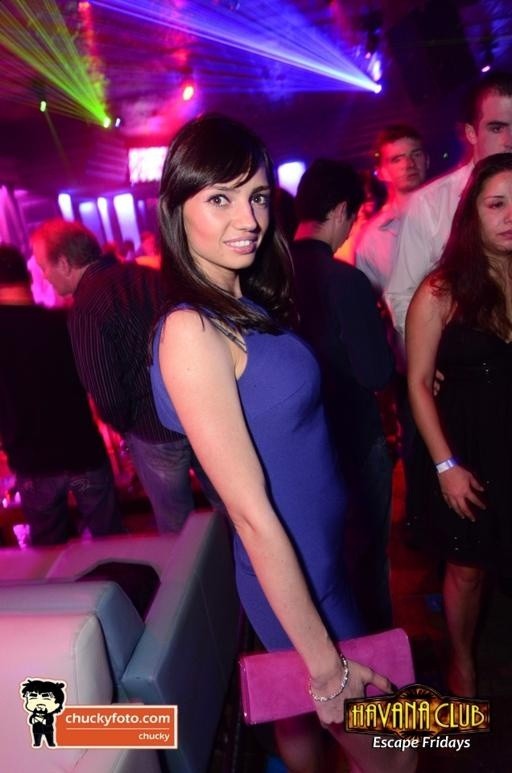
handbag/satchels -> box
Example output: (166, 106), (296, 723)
(237, 628), (417, 725)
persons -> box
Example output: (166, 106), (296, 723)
(0, 67), (512, 773)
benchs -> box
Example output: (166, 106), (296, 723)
(0, 508), (243, 773)
